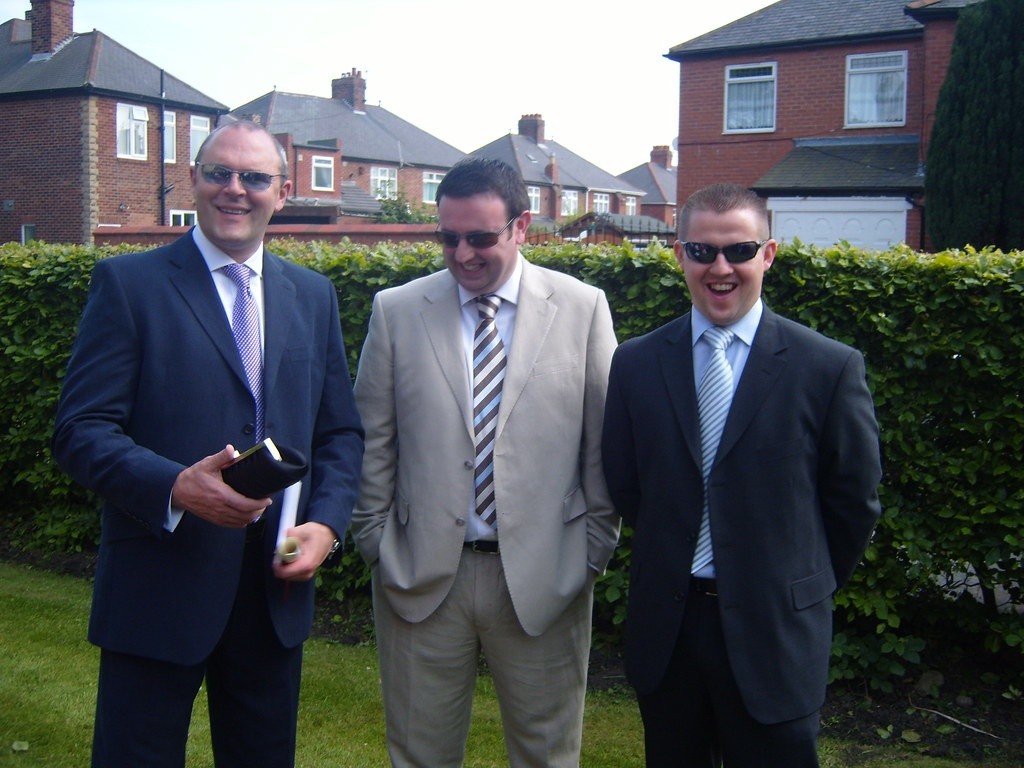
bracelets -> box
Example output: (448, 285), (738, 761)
(329, 539), (341, 560)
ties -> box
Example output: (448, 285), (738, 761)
(222, 264), (266, 522)
(473, 294), (507, 527)
(690, 327), (738, 575)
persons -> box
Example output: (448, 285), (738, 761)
(49, 119), (365, 768)
(600, 184), (883, 768)
(351, 157), (624, 768)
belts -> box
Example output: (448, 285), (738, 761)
(691, 576), (719, 596)
(463, 540), (500, 554)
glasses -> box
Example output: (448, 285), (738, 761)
(194, 162), (287, 191)
(682, 240), (769, 264)
(435, 216), (517, 248)
